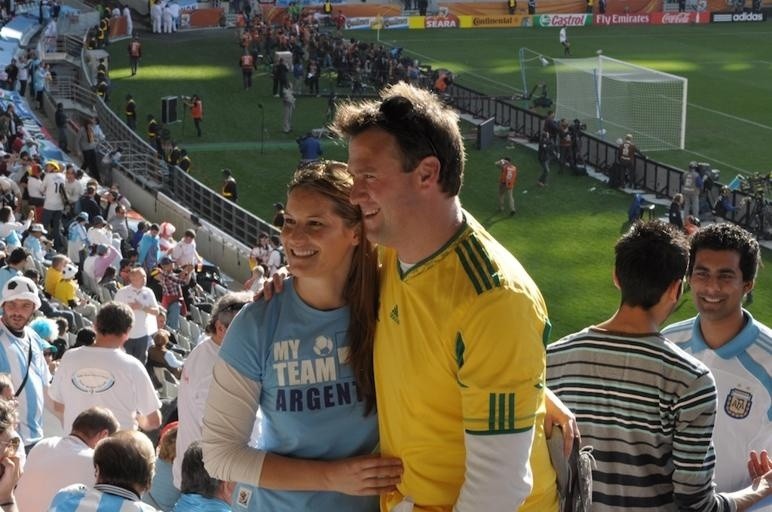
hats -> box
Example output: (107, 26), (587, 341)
(46, 160), (60, 172)
(25, 138), (35, 145)
(221, 168), (231, 176)
(273, 202), (283, 210)
(31, 164), (44, 176)
(160, 257), (176, 265)
(0, 276), (41, 313)
(32, 212), (139, 280)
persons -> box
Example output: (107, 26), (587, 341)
(659, 224), (771, 510)
(252, 81), (564, 511)
(544, 218), (771, 510)
(218, 3), (772, 302)
(0, 0), (283, 512)
(204, 159), (582, 512)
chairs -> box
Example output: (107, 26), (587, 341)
(3, 234), (260, 390)
(0, 0), (115, 186)
